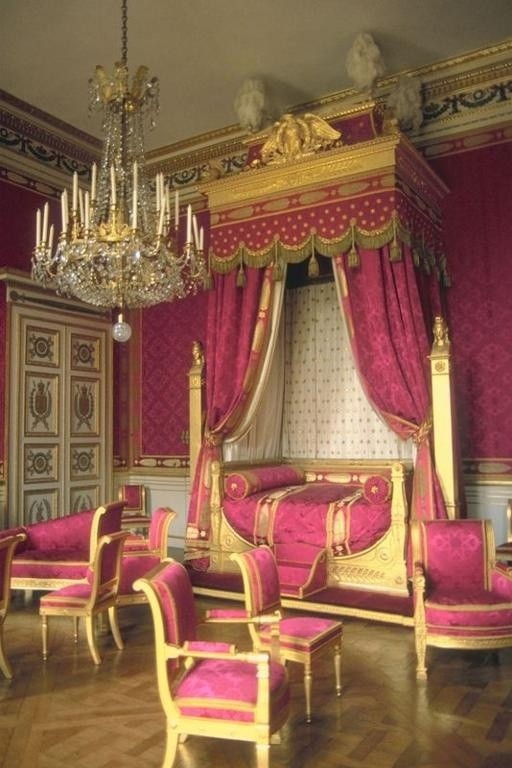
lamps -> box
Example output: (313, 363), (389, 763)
(30, 0), (205, 343)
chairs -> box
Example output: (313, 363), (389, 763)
(495, 499), (512, 565)
(410, 518), (512, 679)
(229, 543), (344, 722)
(132, 559), (290, 767)
(1, 485), (177, 679)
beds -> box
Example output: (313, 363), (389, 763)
(182, 110), (455, 598)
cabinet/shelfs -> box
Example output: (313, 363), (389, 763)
(1, 266), (112, 528)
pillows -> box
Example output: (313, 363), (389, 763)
(225, 465), (302, 499)
(363, 471), (392, 503)
